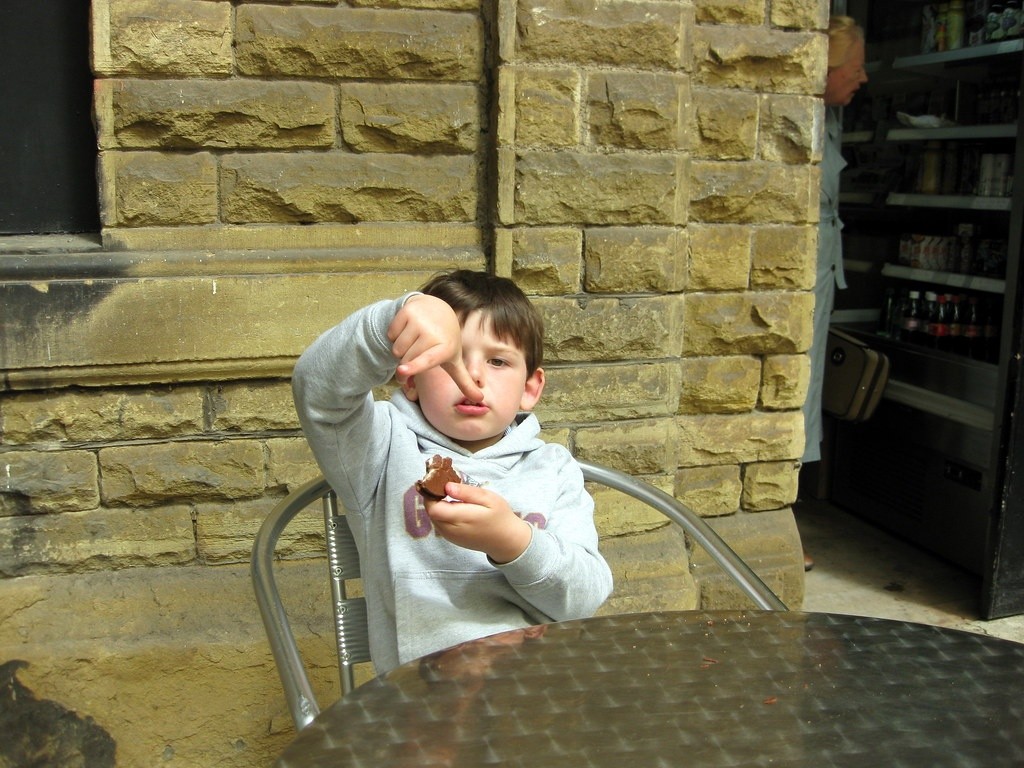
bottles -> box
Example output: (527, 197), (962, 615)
(921, 0), (1024, 54)
(885, 290), (982, 336)
(921, 142), (1009, 197)
(976, 90), (1021, 127)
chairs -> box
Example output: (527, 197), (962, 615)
(250, 455), (791, 736)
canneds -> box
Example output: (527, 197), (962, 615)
(975, 153), (1012, 197)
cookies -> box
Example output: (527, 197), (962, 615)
(415, 456), (462, 500)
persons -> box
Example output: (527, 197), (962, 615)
(791, 14), (869, 571)
(290, 268), (614, 679)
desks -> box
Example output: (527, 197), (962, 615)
(274, 610), (1024, 768)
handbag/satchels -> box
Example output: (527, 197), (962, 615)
(821, 327), (889, 420)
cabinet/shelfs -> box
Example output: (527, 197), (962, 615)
(830, 37), (1024, 622)
